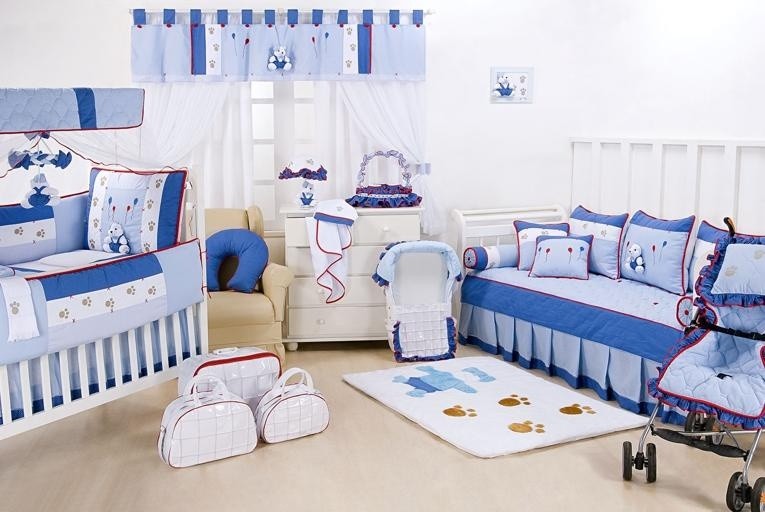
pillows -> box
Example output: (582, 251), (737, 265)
(528, 234), (595, 280)
(567, 205), (630, 279)
(619, 210), (698, 295)
(688, 219), (764, 294)
(513, 218), (570, 271)
(206, 229), (269, 294)
(81, 164), (188, 256)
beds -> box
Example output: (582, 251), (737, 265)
(0, 162), (210, 439)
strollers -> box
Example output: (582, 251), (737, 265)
(618, 215), (765, 510)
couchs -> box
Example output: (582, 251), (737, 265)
(202, 206), (291, 364)
(450, 141), (765, 426)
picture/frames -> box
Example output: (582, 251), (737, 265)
(489, 64), (533, 104)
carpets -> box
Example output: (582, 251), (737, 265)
(345, 355), (654, 458)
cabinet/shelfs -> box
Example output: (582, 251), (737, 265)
(280, 207), (426, 352)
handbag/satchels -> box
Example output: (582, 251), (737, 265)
(178, 346), (281, 412)
(255, 368), (330, 444)
(157, 376), (258, 468)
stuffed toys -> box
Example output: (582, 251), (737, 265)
(266, 45), (292, 71)
(20, 172), (59, 209)
(496, 74), (515, 98)
(102, 221), (131, 254)
(624, 243), (645, 276)
(294, 181), (319, 208)
